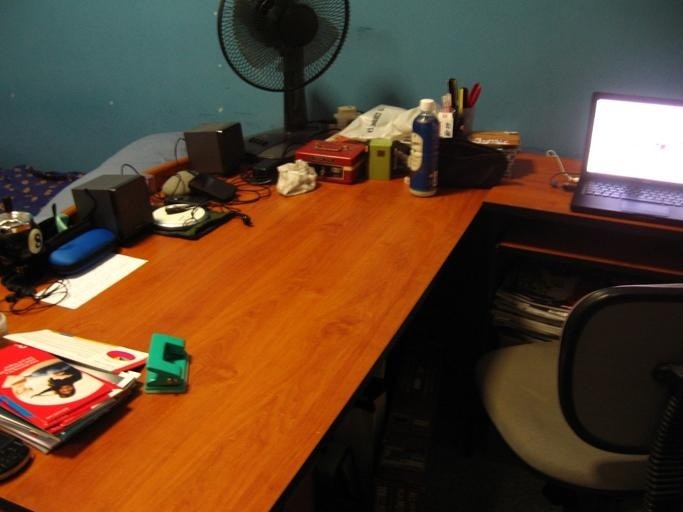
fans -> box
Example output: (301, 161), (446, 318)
(216, 0), (351, 164)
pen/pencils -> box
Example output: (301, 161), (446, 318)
(449, 79), (481, 118)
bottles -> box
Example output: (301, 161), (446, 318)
(409, 98), (440, 198)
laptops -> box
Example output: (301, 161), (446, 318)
(570, 92), (683, 226)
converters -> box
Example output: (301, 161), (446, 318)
(252, 158), (280, 179)
(188, 173), (237, 201)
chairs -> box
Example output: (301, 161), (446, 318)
(477, 282), (683, 512)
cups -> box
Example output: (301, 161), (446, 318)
(455, 108), (473, 135)
(437, 108), (455, 138)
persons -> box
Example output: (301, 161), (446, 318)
(28, 360), (82, 400)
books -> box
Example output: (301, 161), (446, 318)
(485, 263), (601, 346)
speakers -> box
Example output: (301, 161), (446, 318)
(70, 174), (154, 248)
(183, 122), (247, 176)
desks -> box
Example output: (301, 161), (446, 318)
(0, 152), (683, 512)
